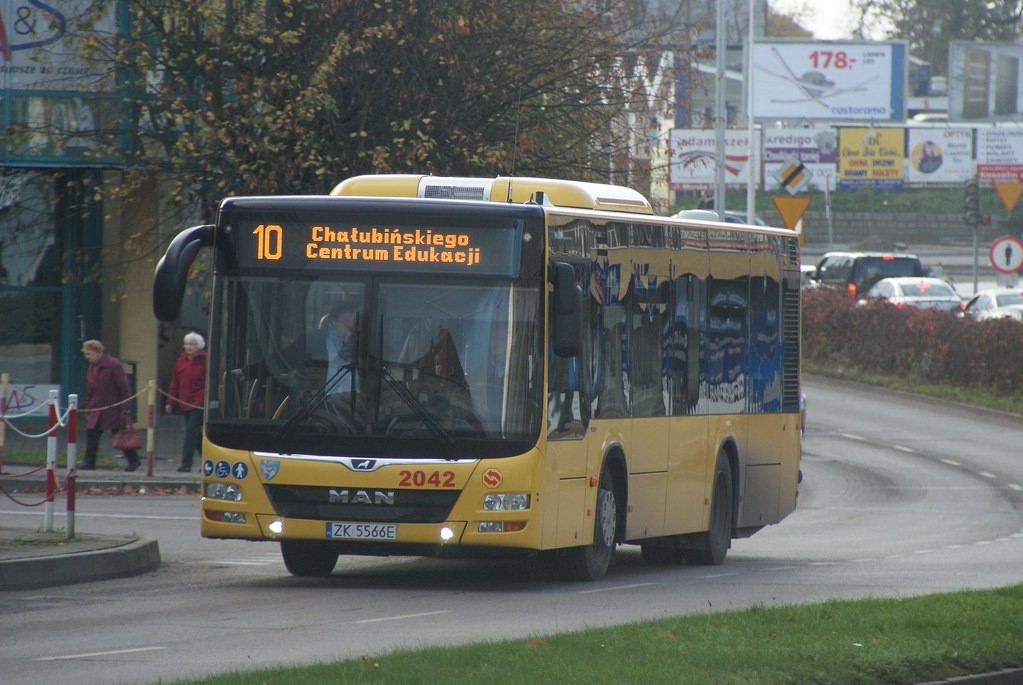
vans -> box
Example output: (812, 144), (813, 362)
(811, 252), (923, 303)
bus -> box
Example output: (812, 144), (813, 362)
(151, 79), (814, 591)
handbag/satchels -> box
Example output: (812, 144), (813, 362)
(112, 409), (144, 450)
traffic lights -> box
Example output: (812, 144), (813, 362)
(980, 214), (991, 227)
(962, 179), (980, 228)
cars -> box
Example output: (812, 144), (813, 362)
(797, 263), (818, 293)
(854, 277), (966, 320)
(721, 210), (769, 228)
(952, 289), (1022, 329)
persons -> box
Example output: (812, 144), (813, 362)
(164, 332), (208, 471)
(230, 300), (361, 395)
(79, 340), (143, 473)
